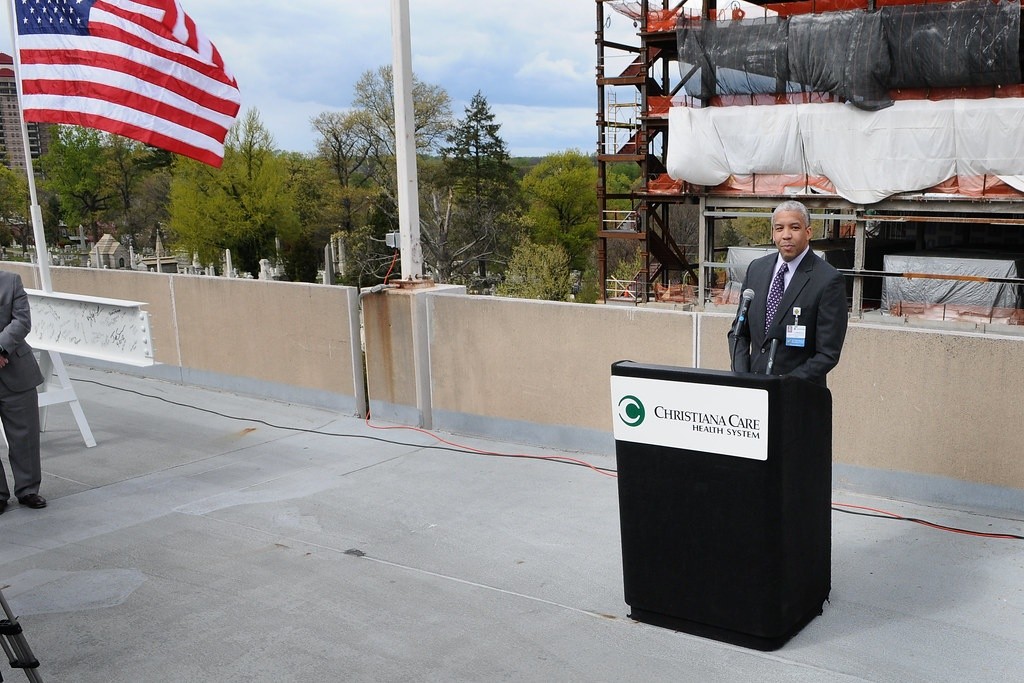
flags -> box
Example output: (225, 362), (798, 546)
(10, 0), (242, 169)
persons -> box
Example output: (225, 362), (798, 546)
(0, 269), (46, 516)
(727, 200), (848, 387)
(788, 326), (792, 332)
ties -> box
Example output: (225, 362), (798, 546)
(765, 263), (788, 337)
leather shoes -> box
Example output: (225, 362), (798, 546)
(0, 500), (7, 515)
(19, 492), (47, 509)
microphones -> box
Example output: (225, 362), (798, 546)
(733, 289), (755, 340)
(765, 324), (786, 374)
